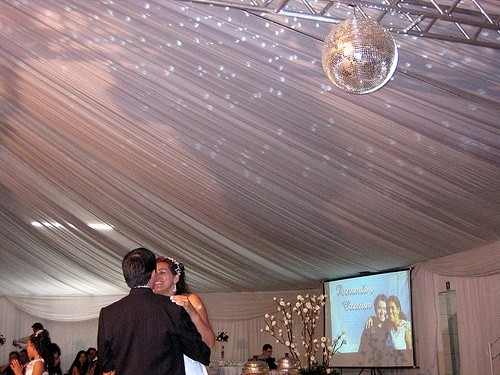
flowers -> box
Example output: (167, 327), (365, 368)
(260, 294), (347, 375)
(216, 332), (229, 360)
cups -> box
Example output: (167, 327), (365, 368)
(210, 359), (244, 366)
(267, 369), (279, 375)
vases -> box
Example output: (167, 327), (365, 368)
(221, 346), (225, 360)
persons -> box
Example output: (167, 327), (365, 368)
(257, 343), (279, 364)
(0, 322), (97, 375)
(358, 294), (412, 352)
(103, 256), (216, 375)
(93, 248), (211, 375)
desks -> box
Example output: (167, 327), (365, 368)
(206, 366), (244, 375)
